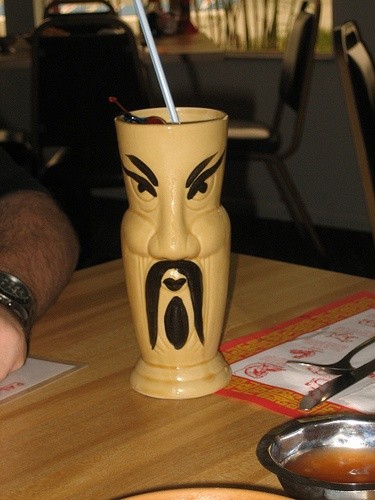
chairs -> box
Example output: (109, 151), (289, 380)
(332, 21), (375, 238)
(227, 0), (327, 260)
(31, 0), (152, 189)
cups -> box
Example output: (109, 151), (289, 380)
(113, 106), (233, 399)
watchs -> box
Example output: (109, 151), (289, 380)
(0, 270), (33, 358)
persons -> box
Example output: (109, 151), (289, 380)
(0, 190), (80, 386)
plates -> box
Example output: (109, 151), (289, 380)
(119, 485), (294, 500)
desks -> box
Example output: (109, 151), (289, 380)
(0, 252), (375, 500)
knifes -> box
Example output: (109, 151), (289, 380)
(300, 359), (375, 411)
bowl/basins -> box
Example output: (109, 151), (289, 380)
(256, 414), (375, 500)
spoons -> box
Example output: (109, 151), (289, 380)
(286, 335), (375, 374)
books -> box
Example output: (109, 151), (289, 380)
(189, 0), (333, 55)
